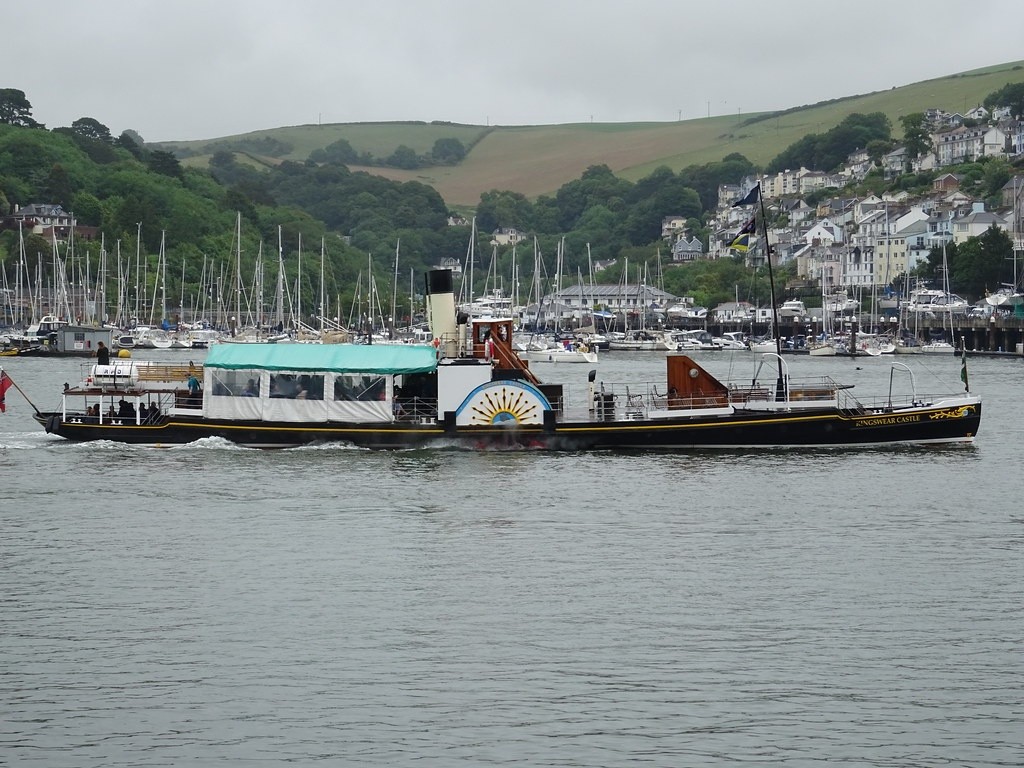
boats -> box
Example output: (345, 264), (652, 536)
(12, 177), (986, 456)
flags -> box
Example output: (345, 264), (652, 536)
(0, 370), (12, 412)
(741, 216), (756, 234)
(726, 233), (749, 251)
(731, 185), (759, 209)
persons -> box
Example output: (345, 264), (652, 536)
(497, 327), (505, 342)
(404, 373), (423, 398)
(257, 375), (402, 401)
(118, 400), (137, 426)
(241, 379), (259, 397)
(146, 402), (160, 423)
(96, 340), (109, 364)
(87, 404), (100, 425)
(110, 406), (118, 417)
(188, 377), (201, 394)
(140, 403), (147, 424)
